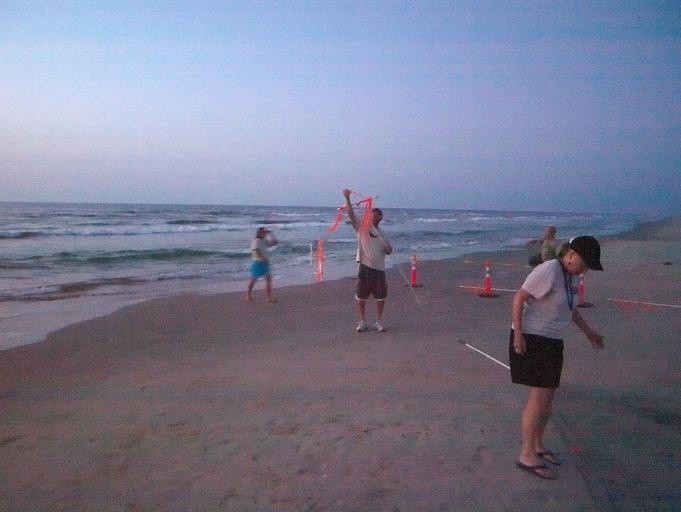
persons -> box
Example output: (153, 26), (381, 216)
(509, 235), (605, 479)
(527, 226), (556, 261)
(247, 227), (278, 303)
(343, 187), (392, 332)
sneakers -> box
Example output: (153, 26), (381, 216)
(375, 320), (383, 332)
(357, 320), (367, 332)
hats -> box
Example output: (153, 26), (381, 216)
(569, 236), (604, 271)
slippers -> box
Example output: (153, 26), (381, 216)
(538, 449), (561, 465)
(519, 459), (556, 479)
